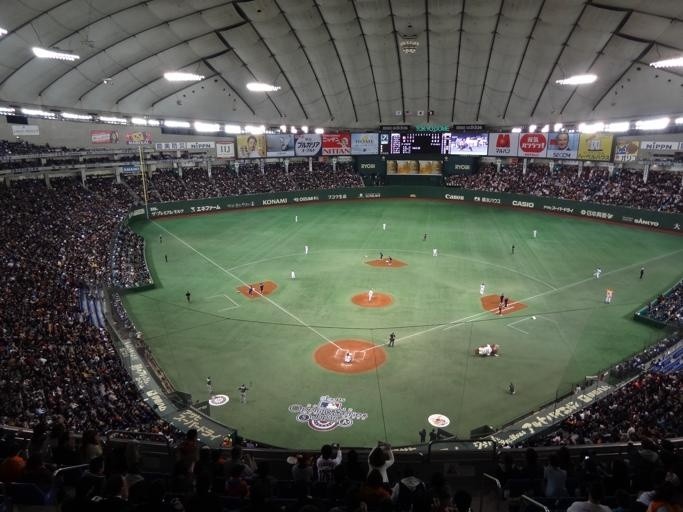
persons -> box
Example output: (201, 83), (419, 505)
(512, 242), (515, 255)
(647, 278), (683, 324)
(371, 174), (385, 185)
(479, 283), (486, 295)
(474, 343), (500, 357)
(499, 292), (509, 316)
(424, 231), (428, 241)
(533, 229), (538, 237)
(383, 223), (387, 230)
(502, 327), (683, 449)
(443, 163), (683, 215)
(369, 288), (374, 302)
(640, 266), (645, 279)
(380, 252), (392, 265)
(433, 248), (438, 256)
(605, 288), (614, 303)
(593, 267), (601, 278)
(2, 422), (683, 512)
(1, 138), (365, 443)
(389, 332), (396, 346)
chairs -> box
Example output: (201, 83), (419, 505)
(3, 178), (151, 421)
(0, 423), (681, 511)
(573, 342), (683, 422)
